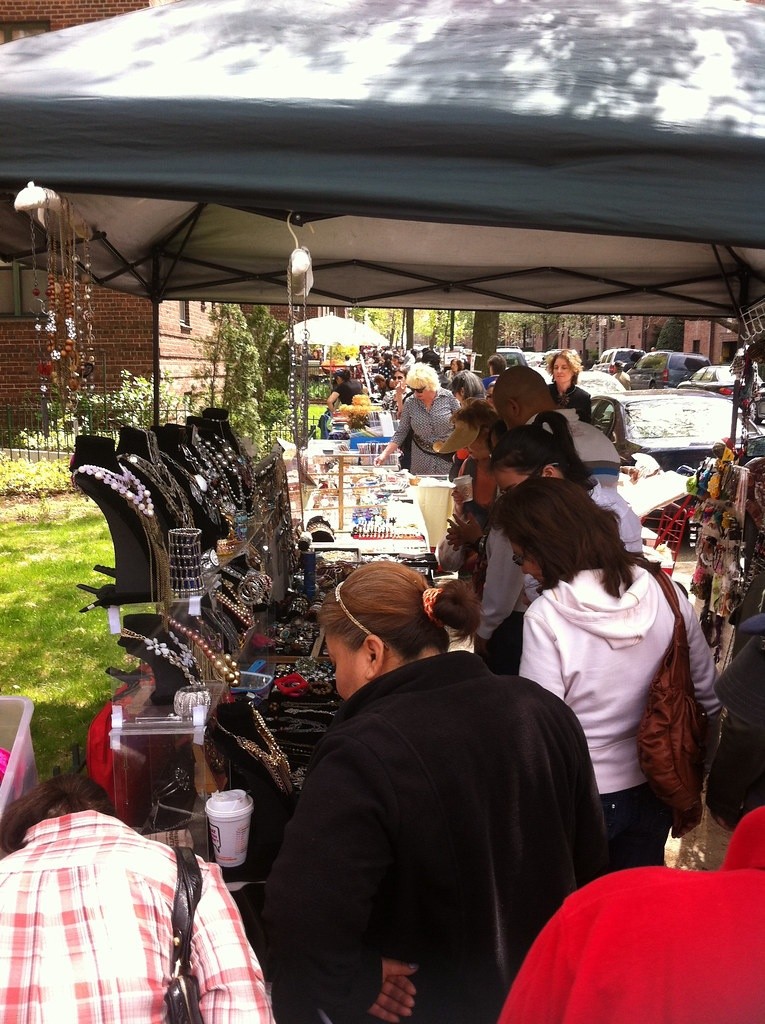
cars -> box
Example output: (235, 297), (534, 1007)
(535, 368), (627, 410)
(677, 364), (765, 425)
(496, 346), (547, 368)
(590, 386), (764, 476)
(412, 344), (472, 369)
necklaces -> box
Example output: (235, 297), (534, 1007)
(77, 415), (336, 794)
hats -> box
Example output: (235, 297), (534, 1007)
(713, 635), (765, 730)
(439, 414), (482, 454)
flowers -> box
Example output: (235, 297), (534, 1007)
(338, 394), (383, 428)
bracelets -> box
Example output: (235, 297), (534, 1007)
(478, 534), (488, 552)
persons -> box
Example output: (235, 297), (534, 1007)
(261, 562), (607, 1024)
(325, 346), (765, 1024)
(1, 774), (278, 1024)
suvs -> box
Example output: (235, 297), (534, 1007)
(626, 351), (713, 389)
(588, 347), (646, 374)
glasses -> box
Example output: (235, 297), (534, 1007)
(393, 375), (403, 380)
(512, 553), (533, 566)
(406, 385), (426, 393)
(500, 462), (559, 495)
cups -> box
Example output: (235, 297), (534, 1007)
(204, 790), (253, 866)
(303, 552), (316, 597)
(454, 474), (474, 503)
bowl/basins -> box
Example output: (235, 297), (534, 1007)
(231, 671), (274, 698)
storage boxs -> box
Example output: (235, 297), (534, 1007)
(0, 697), (39, 819)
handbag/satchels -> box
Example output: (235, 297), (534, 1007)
(637, 570), (708, 839)
(166, 846), (205, 1024)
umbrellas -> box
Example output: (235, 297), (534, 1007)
(283, 312), (389, 392)
